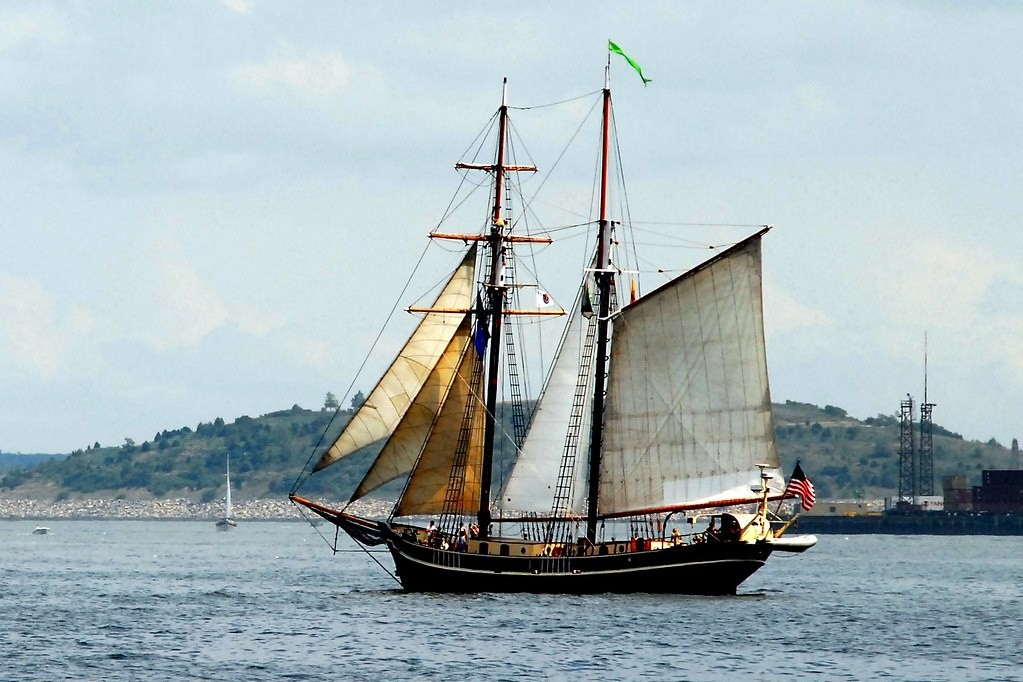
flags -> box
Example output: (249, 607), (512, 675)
(784, 464), (816, 512)
(536, 288), (554, 307)
(609, 40), (653, 88)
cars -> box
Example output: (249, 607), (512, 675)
(947, 510), (1016, 516)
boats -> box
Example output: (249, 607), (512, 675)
(33, 526), (50, 535)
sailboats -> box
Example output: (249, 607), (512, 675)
(216, 453), (237, 531)
(287, 64), (817, 594)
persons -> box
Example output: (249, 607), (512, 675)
(425, 519), (438, 546)
(671, 527), (683, 542)
(459, 522), (479, 552)
(706, 522), (740, 542)
(492, 218), (507, 238)
